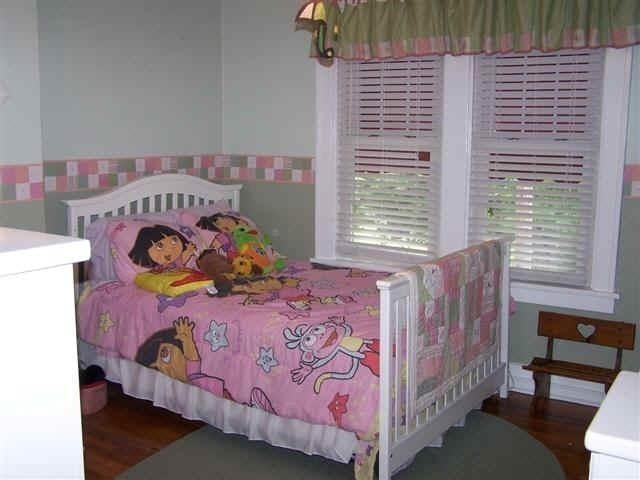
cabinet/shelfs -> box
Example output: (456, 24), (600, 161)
(0, 227), (91, 479)
(584, 371), (640, 480)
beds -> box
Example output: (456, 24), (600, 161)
(59, 174), (509, 480)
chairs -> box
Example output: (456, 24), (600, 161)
(523, 311), (637, 415)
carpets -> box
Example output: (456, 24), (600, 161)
(113, 410), (564, 479)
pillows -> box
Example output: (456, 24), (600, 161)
(175, 213), (275, 263)
(88, 213), (177, 281)
(107, 220), (207, 284)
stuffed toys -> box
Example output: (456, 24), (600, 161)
(233, 224), (284, 274)
(222, 255), (263, 281)
(197, 252), (234, 298)
